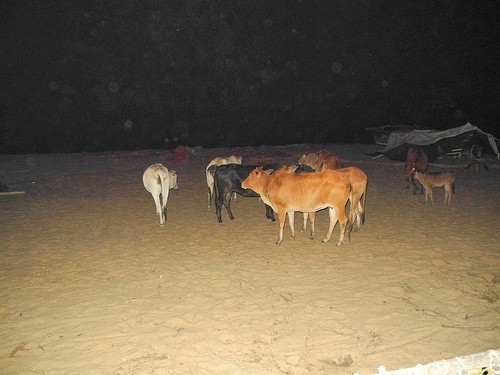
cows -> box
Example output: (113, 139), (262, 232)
(271, 165), (368, 239)
(316, 149), (343, 172)
(213, 163), (316, 223)
(205, 155), (244, 211)
(297, 151), (319, 169)
(142, 163), (178, 226)
(414, 168), (456, 206)
(241, 166), (354, 246)
